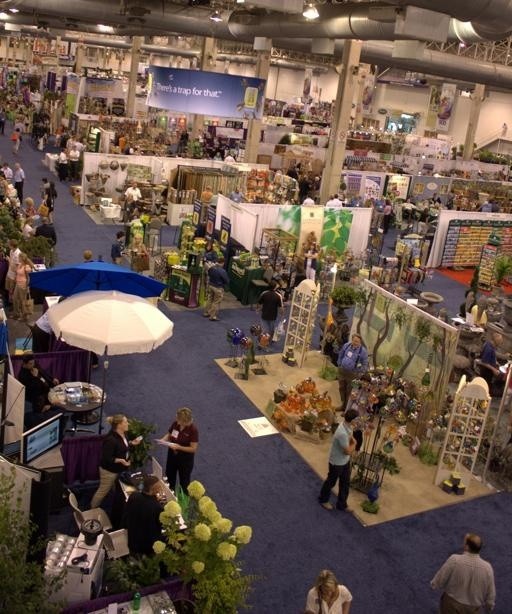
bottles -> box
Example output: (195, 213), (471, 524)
(132, 590), (142, 611)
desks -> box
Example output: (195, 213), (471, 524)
(26, 411), (111, 483)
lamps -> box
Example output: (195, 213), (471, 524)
(302, 1), (319, 22)
(209, 5), (226, 22)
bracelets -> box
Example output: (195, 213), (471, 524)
(129, 440), (133, 446)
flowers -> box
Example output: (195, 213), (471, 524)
(151, 481), (265, 614)
(347, 362), (433, 516)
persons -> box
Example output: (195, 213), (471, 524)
(90, 412), (144, 509)
(2, 65), (512, 400)
(156, 406), (199, 496)
(317, 408), (358, 513)
(334, 332), (369, 411)
(304, 569), (353, 614)
(430, 532), (496, 614)
(342, 373), (374, 451)
(122, 475), (166, 555)
(23, 394), (68, 437)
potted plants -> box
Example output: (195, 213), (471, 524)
(328, 285), (369, 307)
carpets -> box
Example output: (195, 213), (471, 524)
(214, 350), (502, 528)
(119, 242), (258, 314)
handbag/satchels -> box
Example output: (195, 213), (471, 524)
(38, 205), (49, 216)
(277, 321), (285, 336)
(272, 327), (281, 342)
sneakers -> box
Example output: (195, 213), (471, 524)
(345, 507), (354, 513)
(335, 406), (345, 411)
(323, 502), (333, 510)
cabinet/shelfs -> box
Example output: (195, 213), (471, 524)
(261, 228), (299, 302)
(436, 369), (493, 490)
(283, 279), (324, 372)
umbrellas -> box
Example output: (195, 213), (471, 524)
(27, 261), (170, 294)
(47, 289), (175, 434)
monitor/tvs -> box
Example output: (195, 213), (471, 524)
(20, 413), (64, 465)
